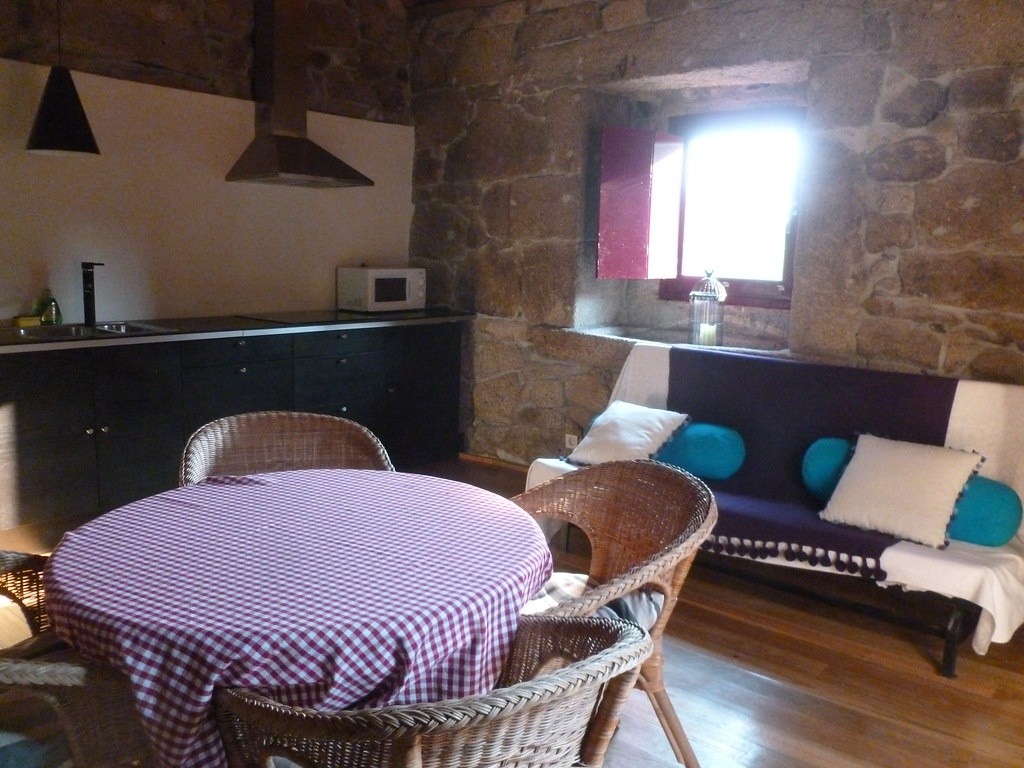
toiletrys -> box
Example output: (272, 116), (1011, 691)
(39, 297), (63, 326)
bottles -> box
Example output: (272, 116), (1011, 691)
(688, 267), (726, 345)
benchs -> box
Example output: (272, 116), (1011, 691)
(525, 341), (1024, 680)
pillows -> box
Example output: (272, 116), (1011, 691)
(801, 438), (1023, 546)
(819, 433), (987, 551)
(560, 399), (691, 467)
(589, 414), (745, 479)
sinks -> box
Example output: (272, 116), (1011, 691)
(89, 323), (155, 335)
(9, 325), (120, 340)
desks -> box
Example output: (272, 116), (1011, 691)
(44, 468), (554, 768)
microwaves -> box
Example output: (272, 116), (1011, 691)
(335, 264), (426, 314)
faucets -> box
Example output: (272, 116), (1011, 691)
(81, 262), (105, 326)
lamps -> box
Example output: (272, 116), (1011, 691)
(25, 0), (101, 156)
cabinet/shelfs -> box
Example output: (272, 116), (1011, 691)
(1, 322), (462, 530)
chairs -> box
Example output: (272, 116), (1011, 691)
(179, 410), (396, 485)
(509, 459), (718, 768)
(0, 550), (154, 768)
(211, 613), (654, 767)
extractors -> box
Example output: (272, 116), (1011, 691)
(224, 0), (375, 190)
